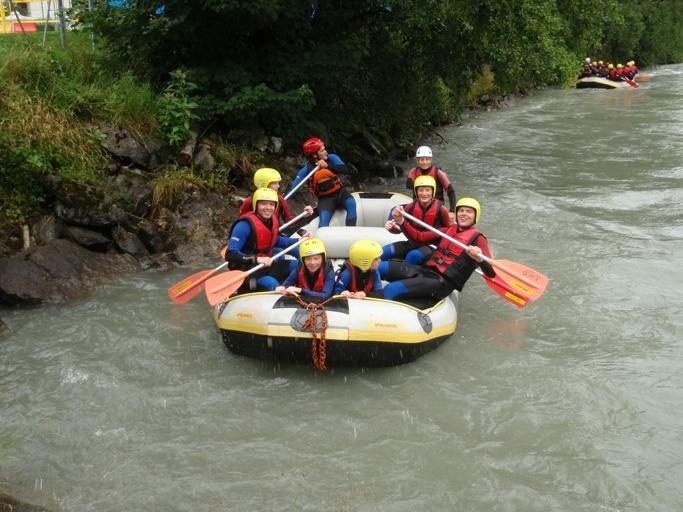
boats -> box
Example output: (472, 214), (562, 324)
(573, 77), (639, 89)
(219, 192), (458, 368)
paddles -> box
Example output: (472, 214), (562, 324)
(398, 210), (553, 303)
(204, 236), (311, 309)
(165, 209), (308, 305)
(614, 67), (650, 87)
(473, 267), (527, 311)
(221, 165), (320, 260)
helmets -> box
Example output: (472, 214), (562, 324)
(349, 240), (384, 271)
(304, 138), (324, 154)
(414, 175), (437, 200)
(253, 188), (278, 213)
(586, 56), (635, 70)
(299, 239), (327, 264)
(455, 197), (480, 224)
(254, 168), (282, 189)
(416, 146), (432, 157)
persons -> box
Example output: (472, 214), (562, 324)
(237, 167), (316, 260)
(273, 237), (333, 297)
(387, 146), (455, 223)
(578, 57), (637, 80)
(332, 242), (383, 299)
(223, 189), (302, 295)
(376, 198), (495, 301)
(382, 175), (451, 264)
(279, 138), (361, 229)
